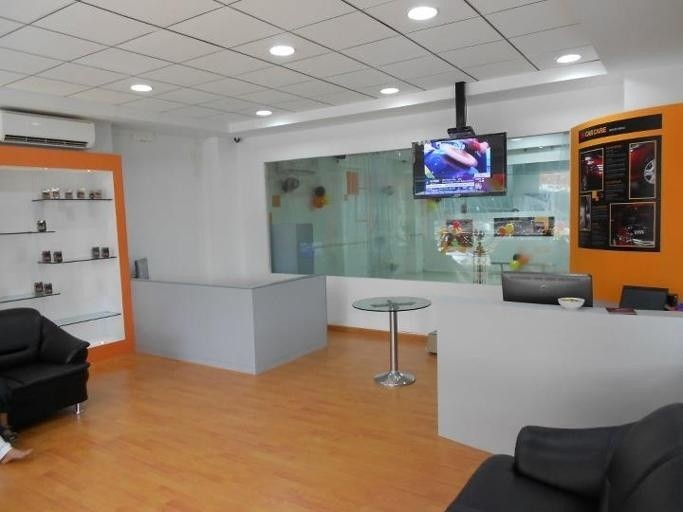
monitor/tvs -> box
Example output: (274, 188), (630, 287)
(134, 256), (149, 280)
(499, 270), (595, 308)
(411, 132), (508, 199)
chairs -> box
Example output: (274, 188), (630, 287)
(0, 308), (91, 439)
(440, 402), (682, 511)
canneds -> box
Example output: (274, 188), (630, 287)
(33, 191), (109, 294)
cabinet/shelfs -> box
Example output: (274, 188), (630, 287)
(0, 197), (116, 332)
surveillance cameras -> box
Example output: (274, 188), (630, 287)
(233, 137), (240, 143)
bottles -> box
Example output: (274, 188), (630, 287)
(54, 251), (62, 263)
(44, 283), (52, 294)
(42, 188), (85, 199)
(92, 247), (100, 258)
(34, 281), (43, 292)
(37, 219), (46, 232)
(101, 247), (109, 258)
(41, 250), (51, 263)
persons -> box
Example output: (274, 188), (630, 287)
(0, 434), (35, 465)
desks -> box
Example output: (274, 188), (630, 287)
(352, 295), (432, 388)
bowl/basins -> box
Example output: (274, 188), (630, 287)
(558, 297), (585, 310)
